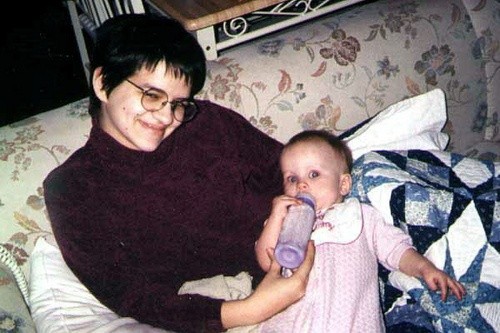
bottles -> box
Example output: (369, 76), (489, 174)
(274, 193), (316, 269)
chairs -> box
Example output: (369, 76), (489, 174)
(66, 0), (145, 92)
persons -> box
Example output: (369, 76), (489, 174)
(42, 13), (315, 333)
(247, 130), (467, 333)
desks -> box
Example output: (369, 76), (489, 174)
(146, 0), (376, 62)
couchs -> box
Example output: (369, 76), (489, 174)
(0, 0), (499, 333)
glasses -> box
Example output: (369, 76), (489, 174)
(124, 77), (202, 123)
